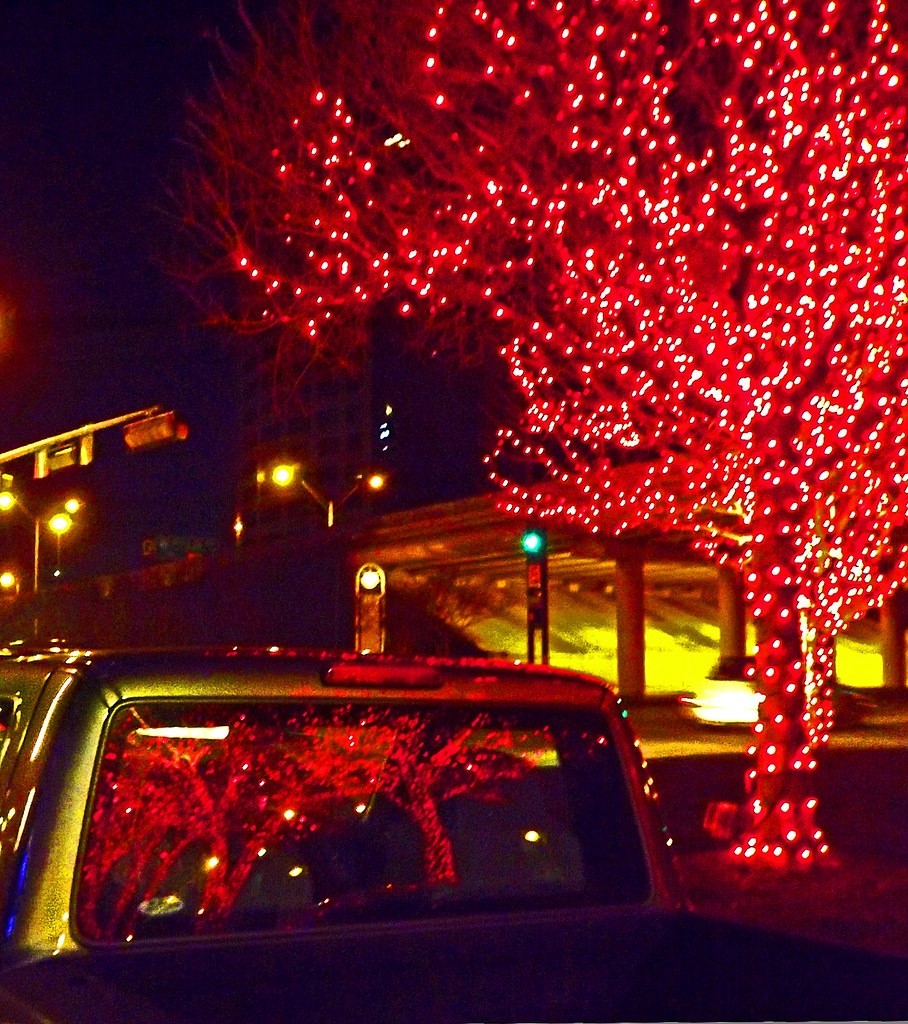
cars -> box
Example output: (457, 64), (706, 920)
(686, 651), (876, 733)
(0, 639), (908, 1023)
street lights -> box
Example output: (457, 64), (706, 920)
(270, 461), (385, 649)
(0, 490), (80, 641)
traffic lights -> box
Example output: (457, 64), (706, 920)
(521, 530), (549, 610)
(355, 562), (387, 659)
